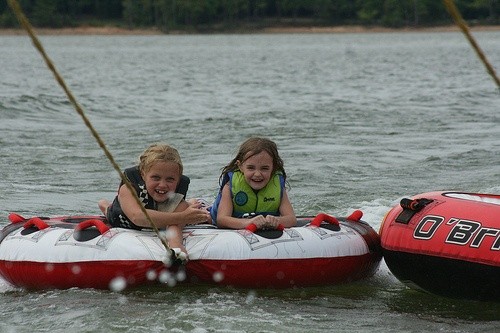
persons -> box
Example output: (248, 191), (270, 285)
(98, 144), (210, 264)
(185, 136), (298, 229)
